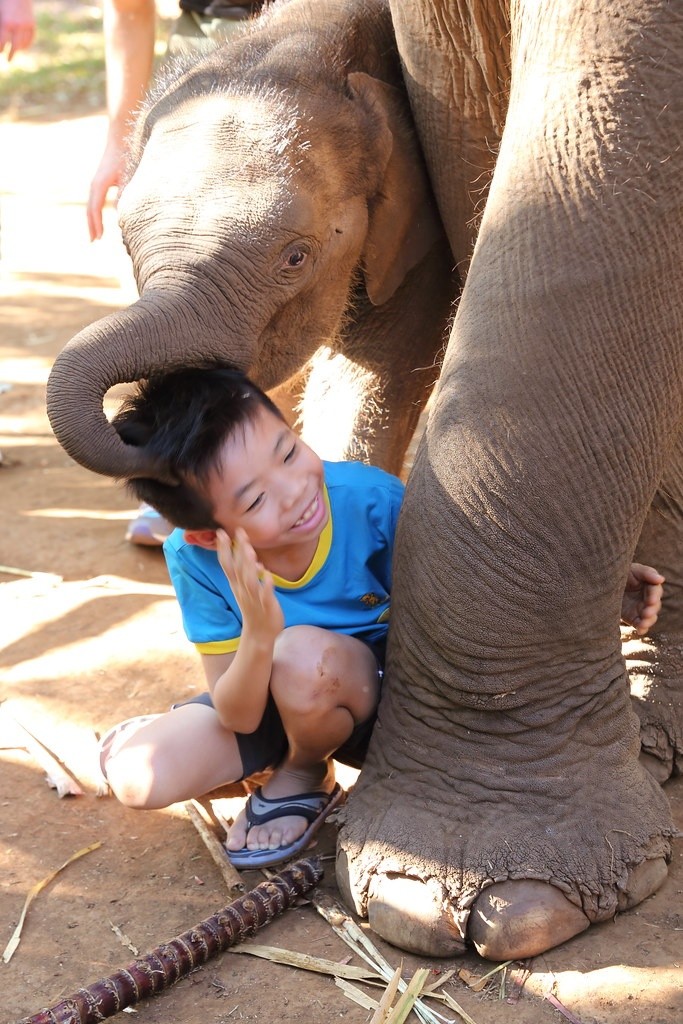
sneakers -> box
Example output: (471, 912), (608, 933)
(127, 500), (176, 546)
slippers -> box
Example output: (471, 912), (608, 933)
(95, 714), (167, 785)
(226, 782), (343, 867)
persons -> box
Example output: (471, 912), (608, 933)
(100, 360), (667, 864)
(86, 2), (269, 546)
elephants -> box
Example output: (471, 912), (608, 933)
(47, 1), (683, 960)
